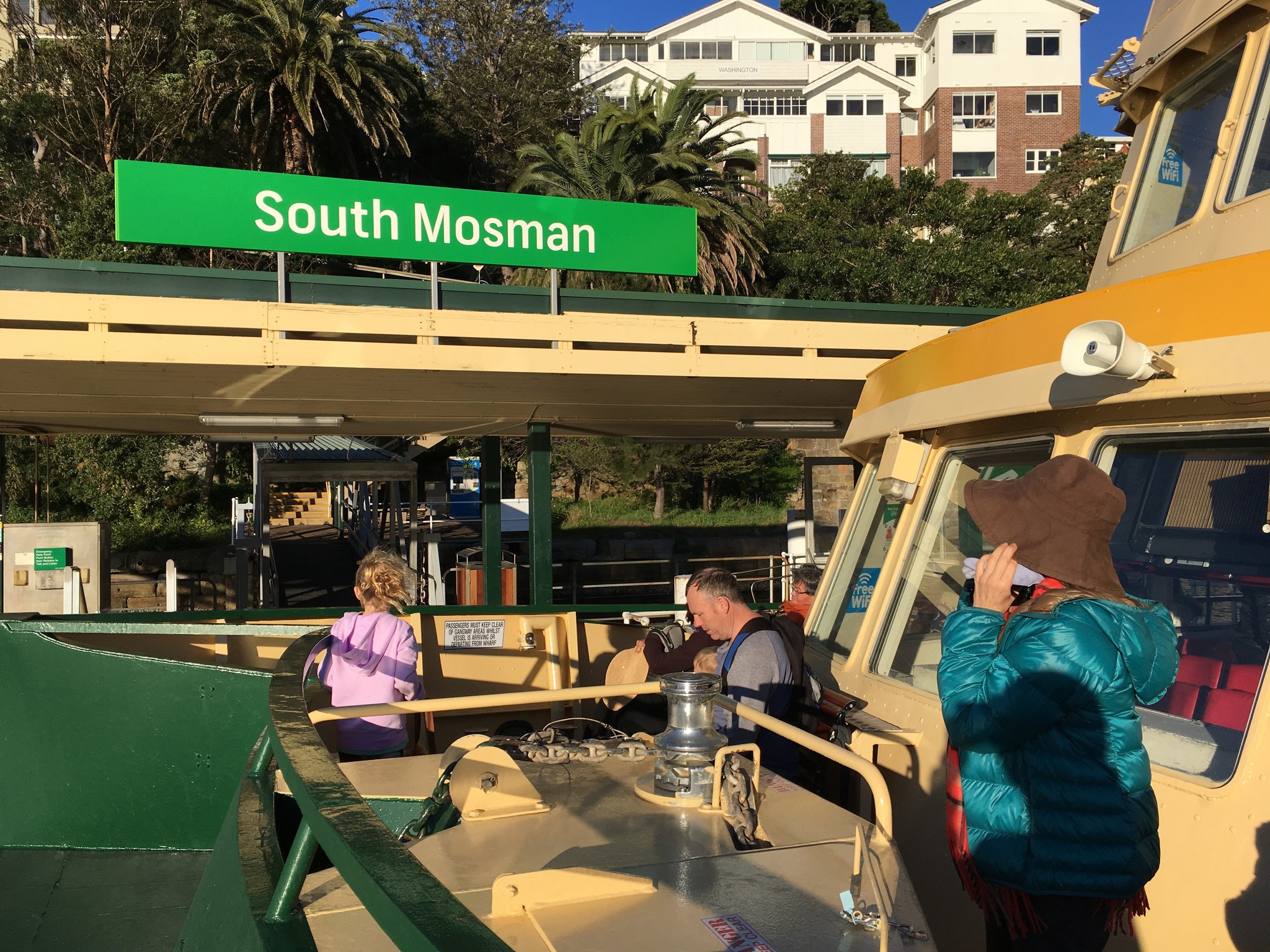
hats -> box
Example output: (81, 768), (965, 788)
(963, 454), (1125, 596)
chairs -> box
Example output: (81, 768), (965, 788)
(1139, 639), (1263, 733)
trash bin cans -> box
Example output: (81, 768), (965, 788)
(456, 547), (517, 606)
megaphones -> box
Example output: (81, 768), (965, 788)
(1061, 319), (1164, 382)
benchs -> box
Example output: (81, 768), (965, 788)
(788, 682), (856, 799)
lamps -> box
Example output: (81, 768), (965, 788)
(198, 414), (344, 428)
(736, 420), (839, 431)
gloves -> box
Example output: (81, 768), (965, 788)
(962, 557), (1046, 587)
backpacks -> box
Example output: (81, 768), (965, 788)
(721, 607), (824, 778)
(652, 619), (687, 652)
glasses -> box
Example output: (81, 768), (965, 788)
(790, 583), (808, 594)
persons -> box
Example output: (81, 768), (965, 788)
(316, 546), (423, 764)
(936, 453), (1180, 952)
(583, 563), (824, 788)
